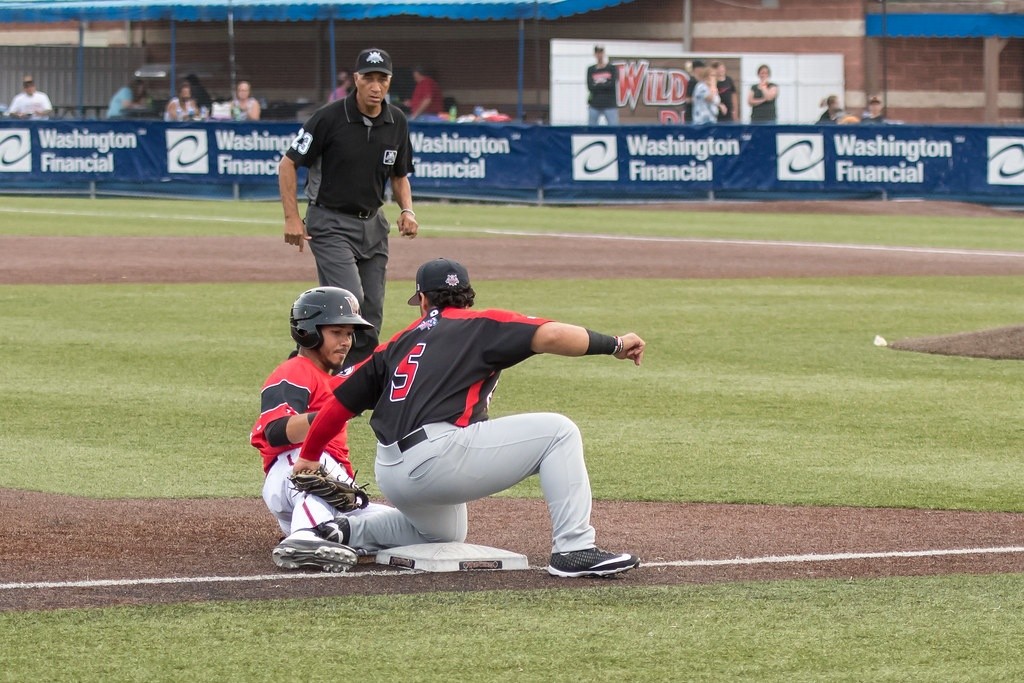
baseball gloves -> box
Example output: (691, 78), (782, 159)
(287, 457), (373, 514)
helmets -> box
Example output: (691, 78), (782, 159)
(289, 286), (374, 350)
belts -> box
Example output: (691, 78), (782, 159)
(398, 429), (428, 454)
(310, 200), (374, 219)
(268, 456), (278, 469)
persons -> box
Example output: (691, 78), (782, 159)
(328, 69), (354, 103)
(291, 257), (645, 578)
(278, 48), (421, 367)
(105, 78), (146, 119)
(860, 96), (885, 123)
(684, 60), (736, 126)
(250, 286), (395, 573)
(8, 76), (52, 121)
(232, 81), (261, 121)
(747, 65), (778, 125)
(586, 46), (619, 126)
(164, 74), (212, 122)
(815, 96), (845, 124)
(402, 67), (444, 120)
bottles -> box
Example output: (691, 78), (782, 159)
(163, 99), (241, 122)
(449, 104), (458, 122)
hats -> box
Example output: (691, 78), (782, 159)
(23, 76), (33, 86)
(868, 96), (881, 104)
(595, 45), (605, 51)
(356, 48), (393, 75)
(408, 258), (469, 306)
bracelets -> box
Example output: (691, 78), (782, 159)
(612, 335), (623, 356)
(401, 209), (415, 216)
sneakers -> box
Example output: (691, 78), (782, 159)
(548, 547), (641, 578)
(272, 531), (360, 573)
(322, 519), (350, 545)
